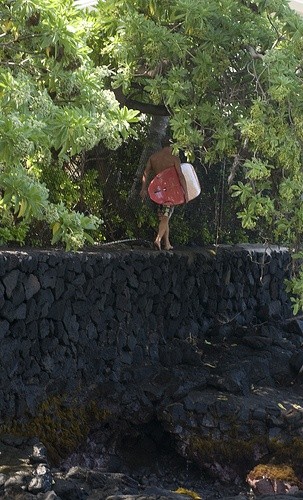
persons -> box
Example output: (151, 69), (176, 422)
(140, 137), (190, 250)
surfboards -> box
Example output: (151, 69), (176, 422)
(148, 163), (200, 205)
